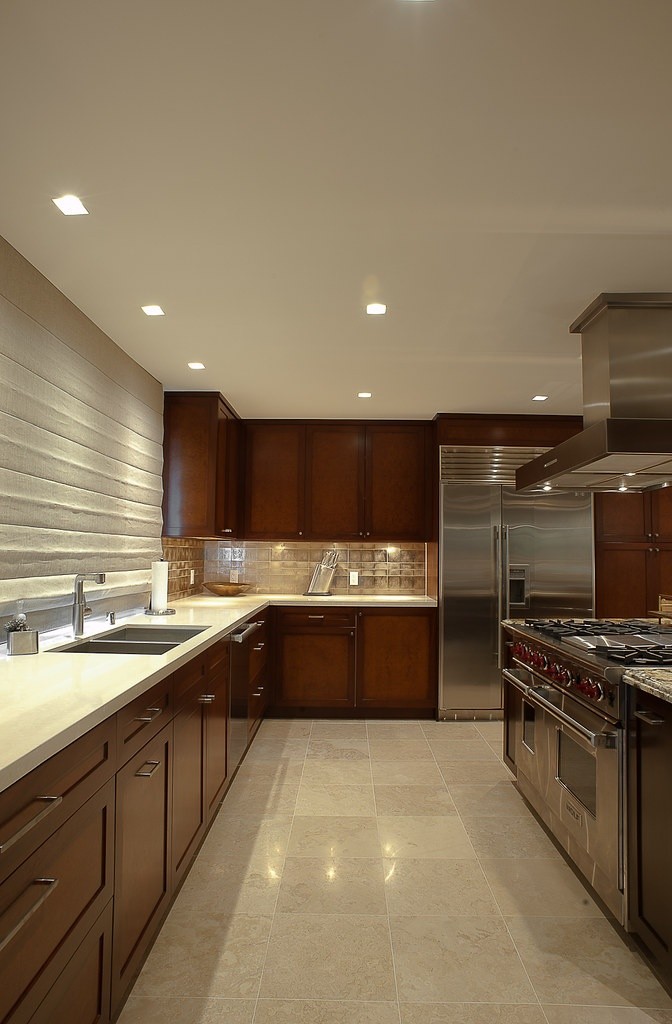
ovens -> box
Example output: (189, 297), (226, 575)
(501, 657), (630, 932)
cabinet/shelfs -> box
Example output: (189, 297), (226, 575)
(357, 607), (438, 720)
(627, 682), (672, 1000)
(173, 633), (231, 901)
(305, 419), (438, 542)
(164, 391), (241, 540)
(240, 418), (305, 542)
(269, 606), (356, 719)
(110, 675), (172, 1024)
(0, 713), (116, 1024)
(595, 488), (672, 619)
(249, 607), (269, 747)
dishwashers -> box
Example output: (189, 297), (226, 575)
(226, 618), (258, 774)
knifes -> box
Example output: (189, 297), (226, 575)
(321, 551), (339, 569)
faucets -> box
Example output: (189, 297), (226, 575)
(72, 573), (106, 636)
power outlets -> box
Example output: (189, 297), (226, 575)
(230, 570), (238, 583)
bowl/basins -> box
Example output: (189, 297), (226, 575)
(202, 582), (251, 596)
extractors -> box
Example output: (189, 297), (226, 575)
(515, 292), (672, 493)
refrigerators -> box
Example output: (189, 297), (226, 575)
(438, 445), (596, 721)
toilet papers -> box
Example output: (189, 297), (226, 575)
(150, 561), (168, 611)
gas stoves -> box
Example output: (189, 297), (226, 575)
(504, 619), (672, 721)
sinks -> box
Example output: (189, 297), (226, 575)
(93, 623), (211, 643)
(44, 643), (179, 662)
